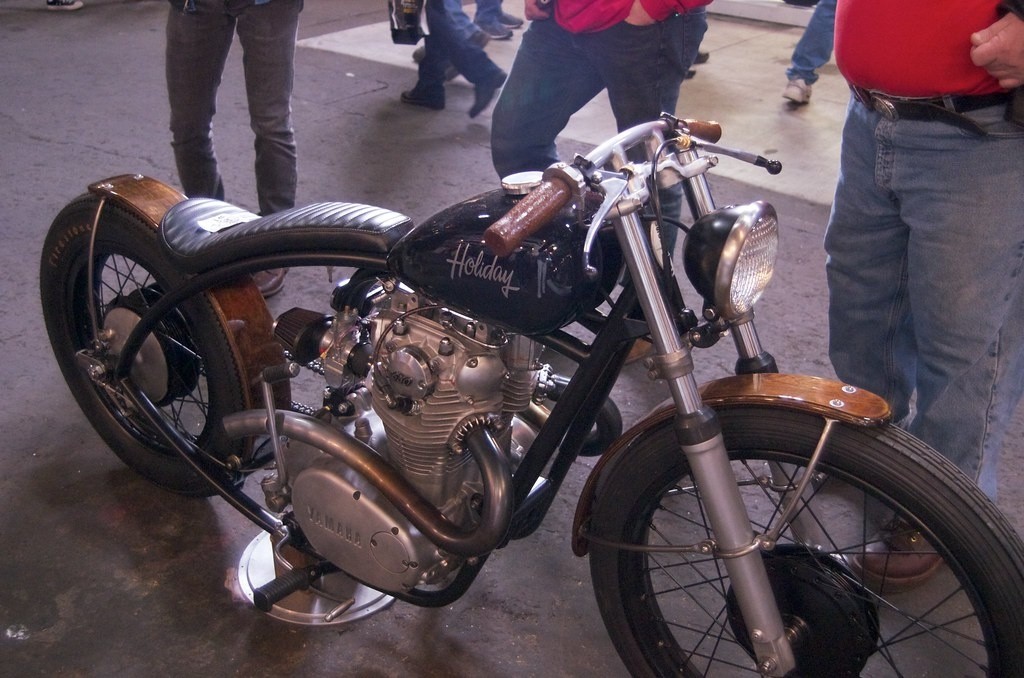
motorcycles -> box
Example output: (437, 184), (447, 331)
(37, 111), (1024, 678)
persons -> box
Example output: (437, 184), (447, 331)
(471, 0), (713, 360)
(783, 1), (835, 104)
(166, 0), (304, 296)
(400, 1), (507, 117)
(473, 0), (523, 39)
(823, 0), (1024, 589)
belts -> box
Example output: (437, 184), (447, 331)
(847, 84), (1016, 138)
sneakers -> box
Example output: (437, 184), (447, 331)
(782, 78), (812, 103)
(473, 12), (524, 39)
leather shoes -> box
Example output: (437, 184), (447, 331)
(253, 267), (290, 297)
(851, 507), (943, 593)
(469, 73), (508, 118)
(400, 90), (445, 110)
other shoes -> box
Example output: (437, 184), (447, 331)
(685, 52), (709, 79)
